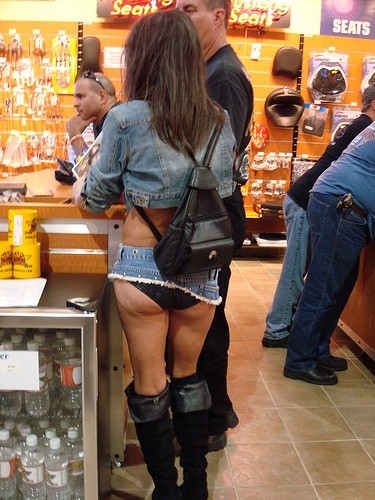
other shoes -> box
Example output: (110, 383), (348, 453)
(226, 407), (239, 428)
(173, 432), (228, 457)
(262, 335), (289, 348)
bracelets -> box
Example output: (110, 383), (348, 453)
(70, 134), (83, 145)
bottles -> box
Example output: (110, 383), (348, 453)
(0, 417), (85, 500)
(0, 328), (82, 416)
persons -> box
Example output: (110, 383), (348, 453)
(262, 85), (375, 347)
(165, 0), (254, 456)
(73, 8), (238, 500)
(67, 69), (122, 166)
(284, 121), (375, 385)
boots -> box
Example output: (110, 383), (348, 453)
(170, 374), (213, 500)
(125, 379), (182, 500)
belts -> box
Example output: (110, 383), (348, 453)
(352, 204), (367, 221)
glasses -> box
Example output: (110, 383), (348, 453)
(83, 70), (105, 91)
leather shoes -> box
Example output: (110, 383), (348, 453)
(316, 355), (348, 372)
(283, 365), (338, 385)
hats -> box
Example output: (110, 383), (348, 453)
(361, 84), (375, 106)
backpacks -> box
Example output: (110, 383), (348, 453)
(131, 120), (236, 282)
